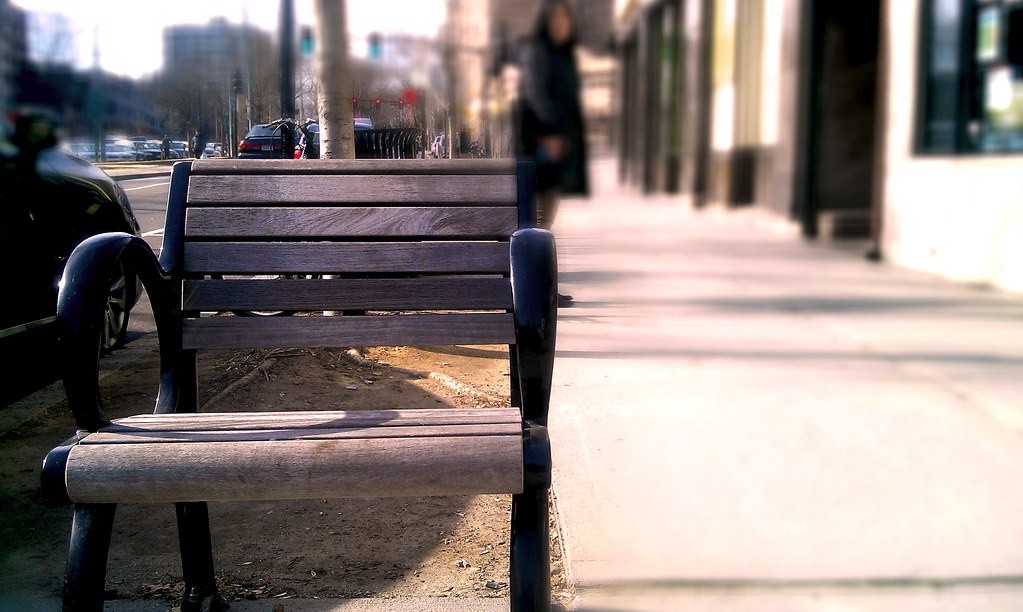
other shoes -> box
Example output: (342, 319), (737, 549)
(558, 293), (574, 308)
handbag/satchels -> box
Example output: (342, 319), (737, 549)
(200, 151), (207, 159)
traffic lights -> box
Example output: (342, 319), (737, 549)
(353, 98), (404, 110)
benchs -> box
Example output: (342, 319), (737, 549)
(39, 157), (559, 611)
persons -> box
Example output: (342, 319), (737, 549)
(519, 0), (591, 308)
(191, 129), (205, 160)
(162, 134), (172, 159)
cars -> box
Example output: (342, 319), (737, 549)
(0, 147), (143, 355)
(431, 136), (460, 159)
(238, 116), (374, 159)
(59, 136), (226, 161)
